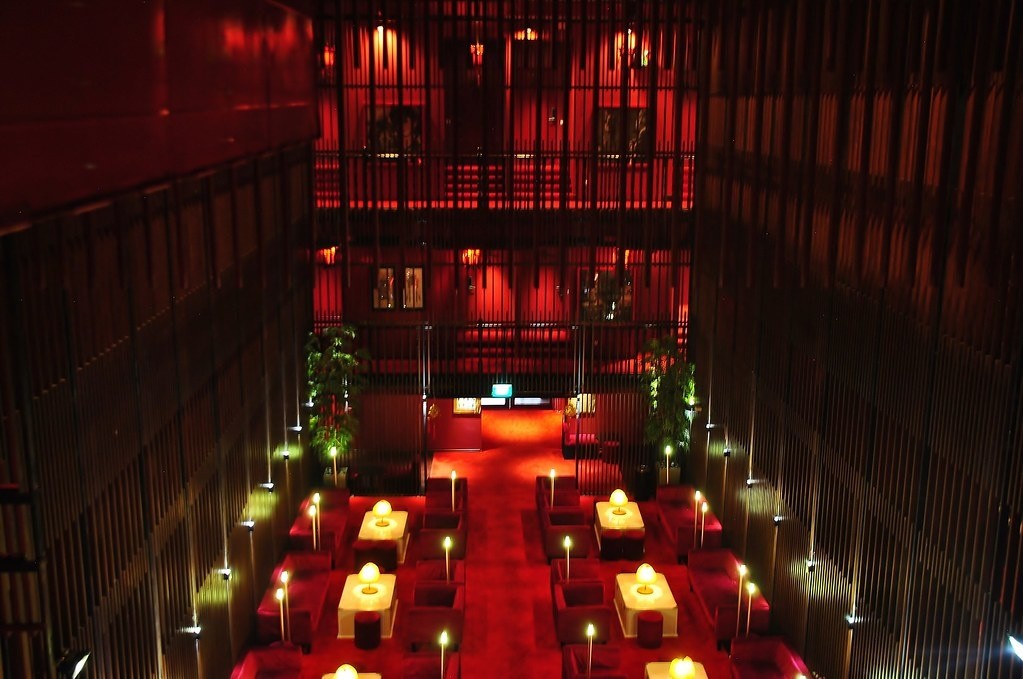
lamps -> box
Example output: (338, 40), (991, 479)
(58, 650), (92, 679)
(669, 655), (695, 679)
(334, 664), (358, 679)
(636, 562), (656, 595)
(609, 488), (629, 515)
(359, 562), (380, 594)
(373, 500), (392, 526)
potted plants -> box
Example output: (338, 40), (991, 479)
(302, 324), (365, 487)
(639, 335), (699, 485)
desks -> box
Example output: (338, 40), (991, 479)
(593, 502), (647, 556)
(645, 660), (708, 679)
(336, 574), (398, 638)
(613, 572), (679, 639)
(357, 510), (411, 563)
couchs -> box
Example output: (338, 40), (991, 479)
(562, 420), (599, 458)
(230, 472), (812, 679)
